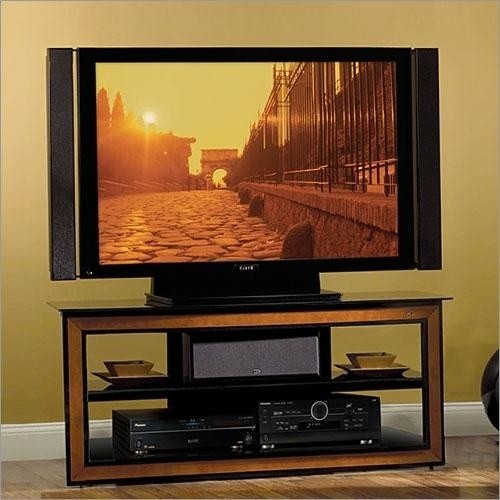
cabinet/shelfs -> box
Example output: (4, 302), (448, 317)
(46, 291), (454, 486)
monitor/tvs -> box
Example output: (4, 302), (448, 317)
(76, 47), (415, 308)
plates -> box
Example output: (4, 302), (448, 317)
(92, 371), (169, 387)
(334, 363), (410, 378)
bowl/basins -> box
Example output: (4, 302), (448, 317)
(346, 351), (397, 370)
(102, 359), (154, 376)
